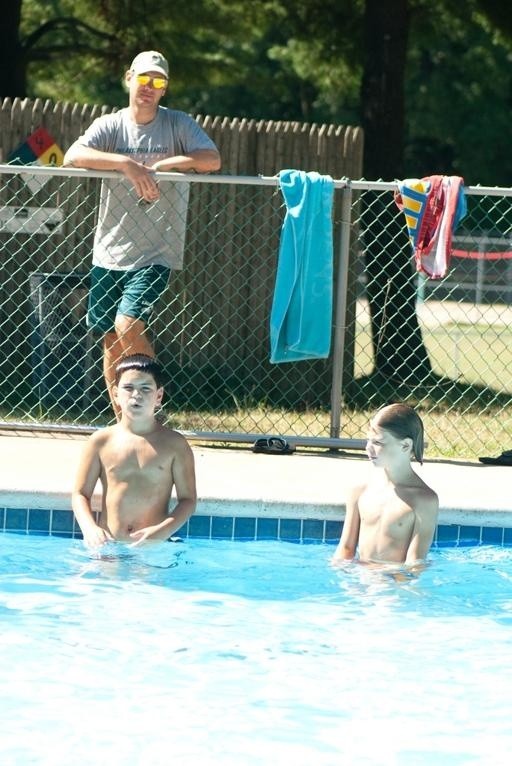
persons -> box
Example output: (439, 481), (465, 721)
(332, 404), (439, 566)
(73, 353), (197, 550)
(63, 50), (222, 423)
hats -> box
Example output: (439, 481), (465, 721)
(130, 50), (169, 79)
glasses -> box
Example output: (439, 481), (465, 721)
(135, 74), (168, 90)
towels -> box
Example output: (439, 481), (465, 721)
(266, 167), (334, 367)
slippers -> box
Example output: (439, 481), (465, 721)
(251, 436), (297, 456)
